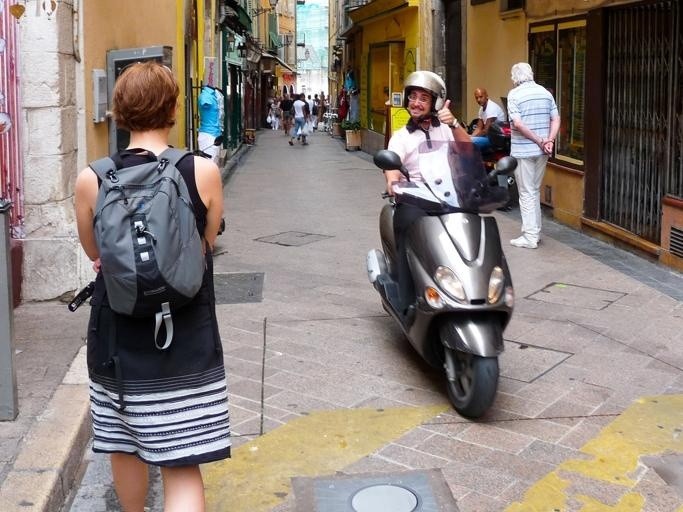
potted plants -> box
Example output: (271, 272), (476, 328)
(341, 119), (362, 151)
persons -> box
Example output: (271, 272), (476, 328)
(469, 87), (506, 151)
(336, 85), (347, 121)
(198, 84), (222, 158)
(382, 87), (390, 105)
(506, 61), (561, 250)
(213, 87), (226, 132)
(73, 62), (231, 511)
(384, 70), (477, 327)
(264, 91), (329, 146)
(344, 64), (357, 96)
(348, 86), (359, 122)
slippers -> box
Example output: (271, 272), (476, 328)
(301, 142), (309, 145)
(288, 140), (294, 147)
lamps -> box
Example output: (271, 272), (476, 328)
(297, 48), (310, 62)
(278, 30), (293, 47)
(252, 0), (278, 17)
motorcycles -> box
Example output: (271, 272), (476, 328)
(359, 134), (524, 421)
(460, 117), (519, 213)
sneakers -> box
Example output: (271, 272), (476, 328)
(510, 235), (538, 249)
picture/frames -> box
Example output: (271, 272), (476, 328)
(392, 92), (402, 107)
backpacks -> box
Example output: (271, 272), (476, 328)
(89, 146), (209, 321)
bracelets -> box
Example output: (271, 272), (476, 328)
(538, 137), (544, 149)
(449, 117), (458, 130)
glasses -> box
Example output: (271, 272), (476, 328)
(407, 93), (431, 104)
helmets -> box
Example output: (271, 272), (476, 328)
(401, 70), (448, 113)
